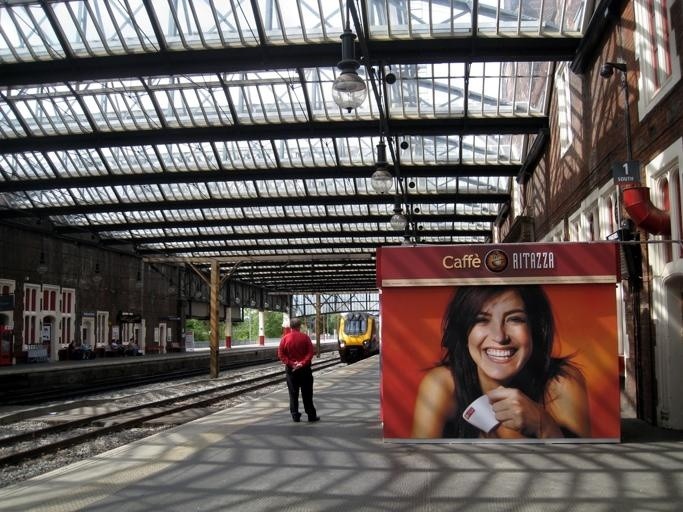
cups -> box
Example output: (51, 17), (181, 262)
(462, 393), (502, 434)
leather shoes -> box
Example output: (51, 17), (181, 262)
(308, 416), (320, 422)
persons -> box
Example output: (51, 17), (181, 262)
(65, 337), (138, 360)
(276, 317), (321, 423)
(412, 286), (593, 438)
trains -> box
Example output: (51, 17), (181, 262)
(338, 313), (379, 363)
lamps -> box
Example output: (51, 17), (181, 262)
(600, 62), (626, 80)
(330, 1), (416, 247)
